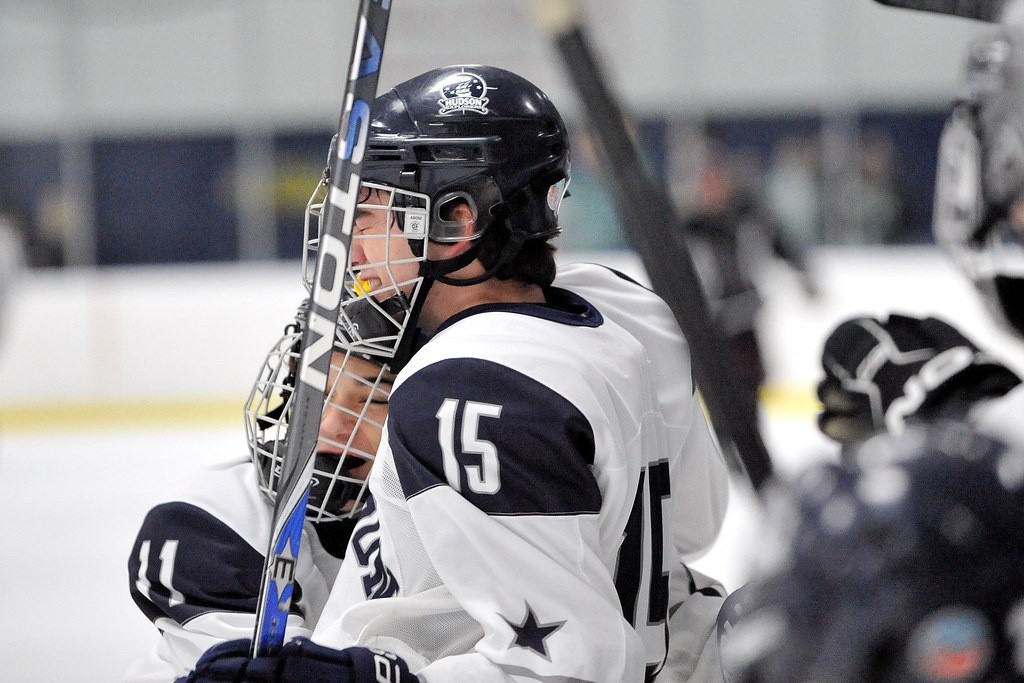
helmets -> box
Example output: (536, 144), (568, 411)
(933, 26), (1024, 340)
(242, 290), (428, 524)
(768, 452), (1024, 683)
(301, 66), (573, 360)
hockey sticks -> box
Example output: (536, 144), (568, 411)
(247, 0), (396, 664)
(531, 2), (780, 499)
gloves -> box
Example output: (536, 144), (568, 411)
(818, 314), (1023, 443)
(173, 636), (420, 683)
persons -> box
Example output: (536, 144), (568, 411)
(172, 62), (733, 683)
(127, 1), (1024, 683)
